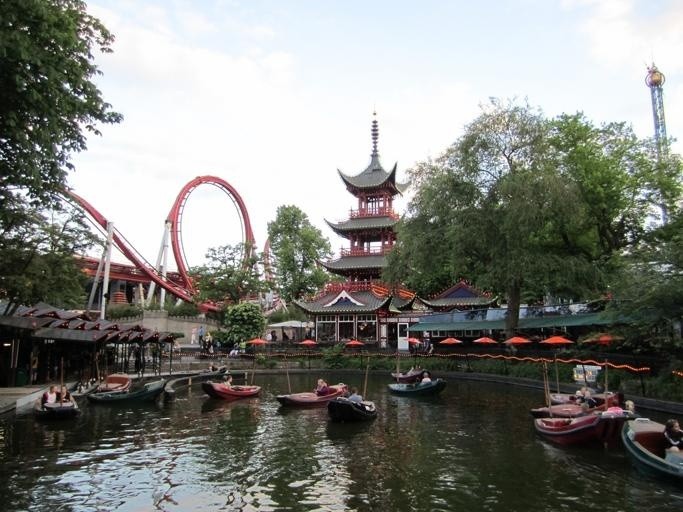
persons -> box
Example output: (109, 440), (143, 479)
(56, 385), (70, 403)
(271, 331), (289, 341)
(421, 372), (431, 384)
(199, 326), (213, 354)
(42, 386), (59, 404)
(26, 346), (143, 388)
(574, 387), (683, 456)
(348, 387), (362, 403)
(210, 366), (232, 386)
(314, 379), (329, 396)
(230, 339), (246, 358)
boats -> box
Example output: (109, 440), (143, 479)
(327, 397), (378, 424)
(391, 369), (426, 379)
(621, 417), (683, 478)
(531, 392), (630, 446)
(95, 371), (132, 394)
(87, 381), (164, 402)
(201, 380), (262, 401)
(388, 378), (447, 395)
(275, 383), (349, 408)
(33, 401), (81, 418)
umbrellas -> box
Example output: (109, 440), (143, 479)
(406, 338), (421, 345)
(502, 337), (532, 344)
(440, 338), (463, 343)
(346, 340), (365, 346)
(472, 337), (498, 343)
(583, 334), (624, 342)
(539, 337), (575, 343)
